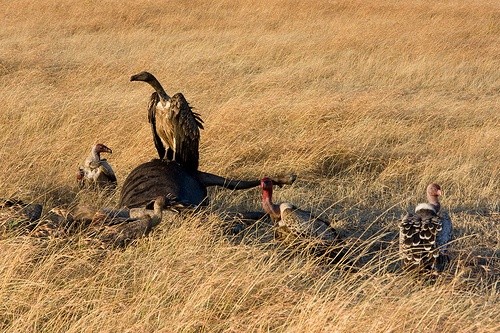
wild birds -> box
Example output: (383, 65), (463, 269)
(99, 196), (171, 251)
(399, 183), (452, 273)
(260, 177), (341, 246)
(76, 143), (118, 197)
(130, 71), (205, 171)
